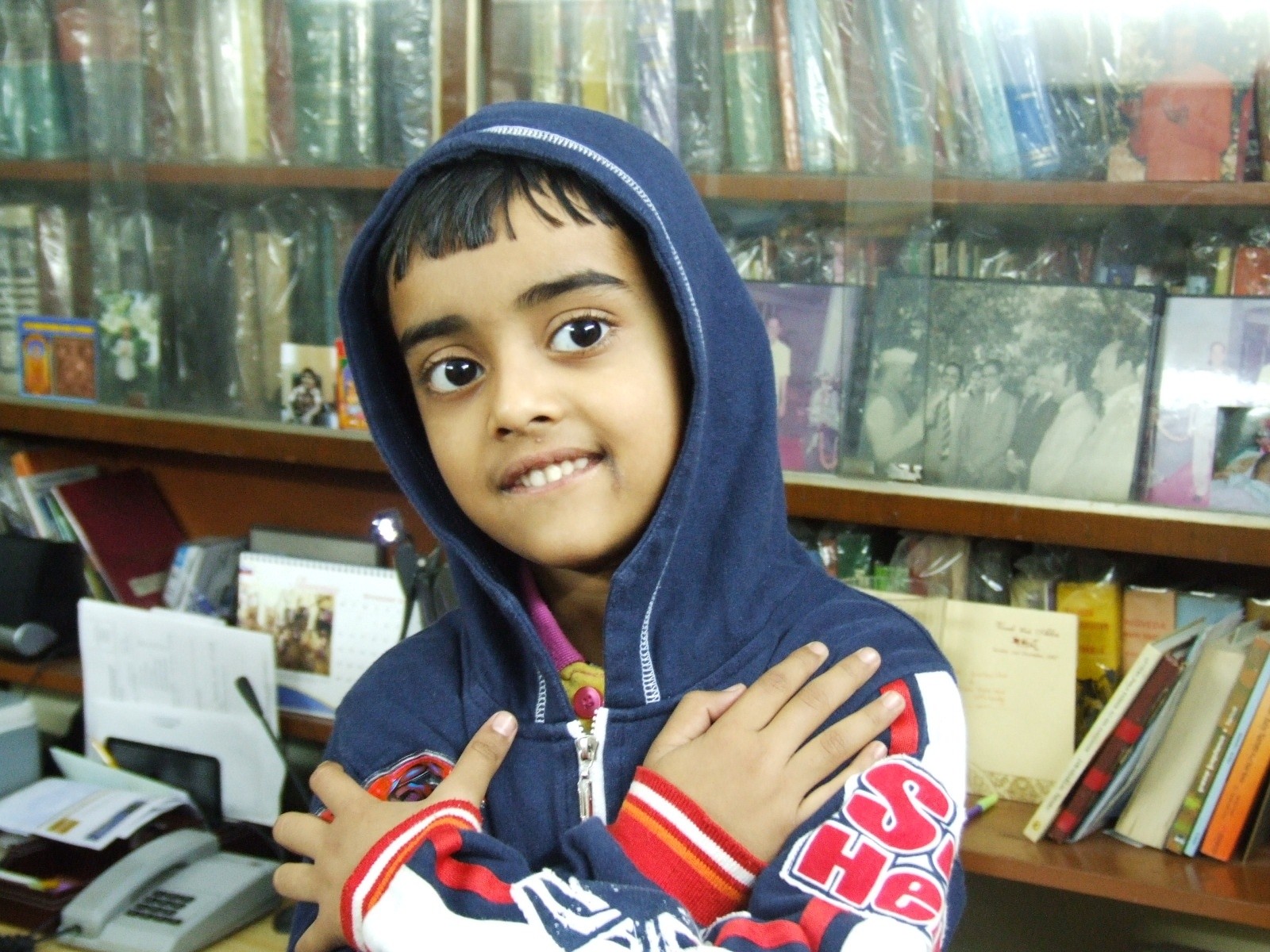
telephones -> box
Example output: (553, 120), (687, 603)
(55, 829), (284, 951)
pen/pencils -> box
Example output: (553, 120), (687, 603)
(966, 793), (999, 823)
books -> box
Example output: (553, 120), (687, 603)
(788, 512), (1270, 864)
(0, 435), (423, 853)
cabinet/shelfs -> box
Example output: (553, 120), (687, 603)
(0, 2), (1270, 952)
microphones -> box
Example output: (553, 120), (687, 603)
(235, 675), (313, 811)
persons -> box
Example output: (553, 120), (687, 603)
(1211, 450), (1270, 513)
(271, 100), (965, 952)
(1130, 16), (1231, 180)
(290, 369), (320, 425)
(862, 335), (1141, 504)
(1194, 343), (1231, 498)
(765, 318), (790, 427)
(806, 370), (840, 456)
